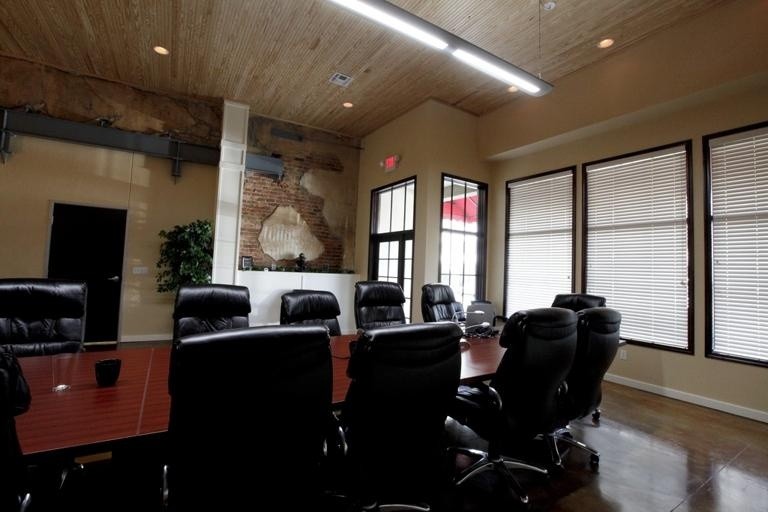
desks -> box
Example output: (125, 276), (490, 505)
(13, 327), (626, 457)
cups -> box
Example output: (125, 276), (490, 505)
(95, 358), (121, 387)
(51, 353), (74, 393)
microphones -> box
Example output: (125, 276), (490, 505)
(451, 310), (485, 322)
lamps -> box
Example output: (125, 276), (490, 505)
(330, 0), (555, 98)
